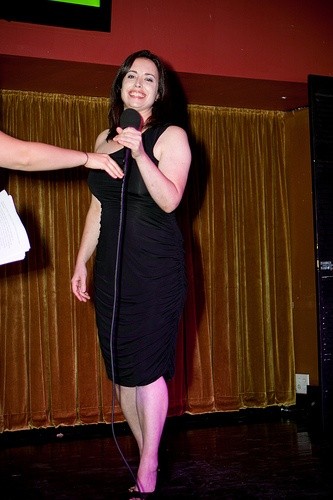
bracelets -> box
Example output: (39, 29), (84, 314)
(80, 152), (88, 168)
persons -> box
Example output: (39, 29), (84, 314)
(0, 130), (124, 180)
(71, 50), (192, 500)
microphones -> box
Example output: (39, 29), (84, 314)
(120, 108), (141, 181)
